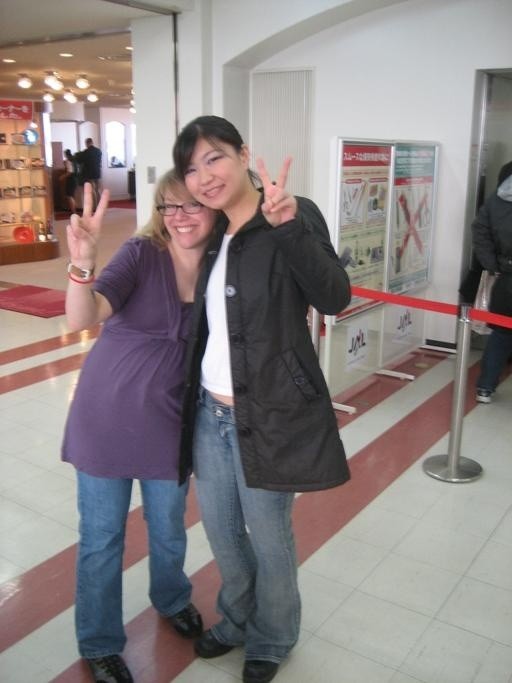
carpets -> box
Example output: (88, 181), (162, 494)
(54, 200), (136, 221)
(0, 281), (66, 319)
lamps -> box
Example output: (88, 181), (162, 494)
(19, 70), (99, 102)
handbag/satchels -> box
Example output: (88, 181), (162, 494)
(470, 268), (500, 336)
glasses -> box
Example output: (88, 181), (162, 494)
(155, 202), (204, 216)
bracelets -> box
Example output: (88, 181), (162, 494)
(68, 272), (95, 284)
(67, 263), (95, 280)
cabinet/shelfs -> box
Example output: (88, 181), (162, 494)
(0, 111), (61, 265)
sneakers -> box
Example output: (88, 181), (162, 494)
(167, 603), (203, 639)
(85, 654), (133, 683)
(241, 660), (278, 682)
(193, 629), (234, 660)
(473, 386), (493, 404)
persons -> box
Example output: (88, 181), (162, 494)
(74, 137), (102, 212)
(457, 159), (512, 303)
(470, 173), (512, 404)
(171, 113), (352, 683)
(58, 149), (78, 216)
(59, 167), (215, 682)
